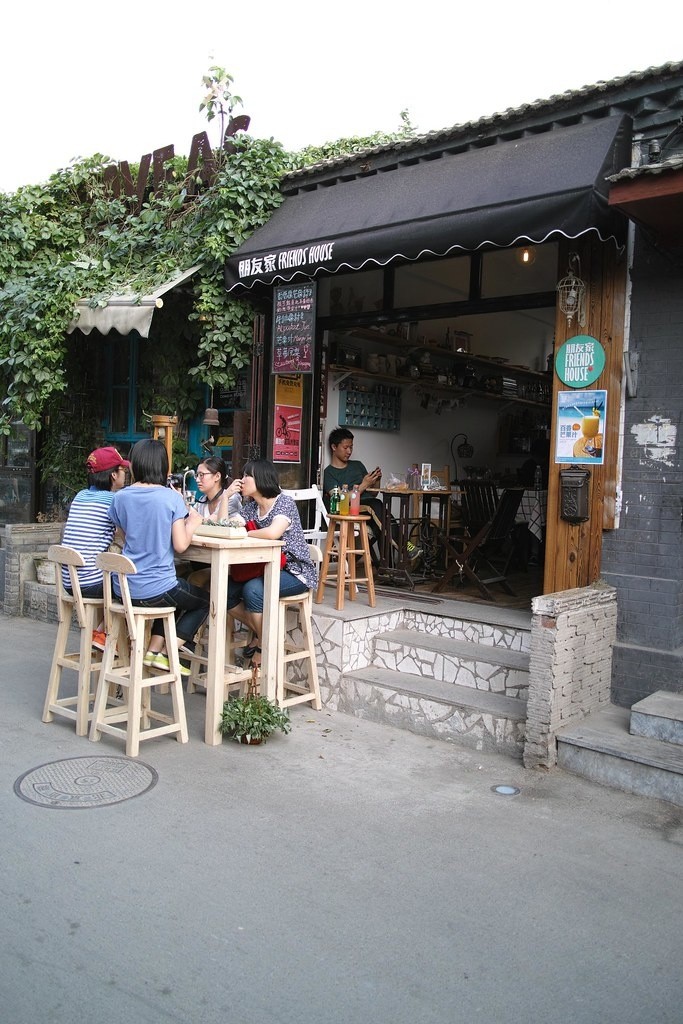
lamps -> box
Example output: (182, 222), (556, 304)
(555, 251), (587, 328)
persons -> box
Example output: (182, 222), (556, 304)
(108, 441), (211, 675)
(191, 459), (241, 591)
(322, 429), (424, 576)
(61, 448), (131, 657)
(218, 460), (318, 668)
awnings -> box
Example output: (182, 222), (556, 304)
(64, 263), (205, 339)
(224, 114), (629, 293)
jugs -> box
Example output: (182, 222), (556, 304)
(368, 353), (420, 380)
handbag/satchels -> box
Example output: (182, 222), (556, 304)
(230, 520), (286, 581)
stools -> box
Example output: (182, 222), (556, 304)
(315, 513), (376, 611)
(189, 546), (321, 715)
(41, 545), (136, 735)
(88, 552), (189, 758)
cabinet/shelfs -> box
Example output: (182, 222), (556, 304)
(330, 325), (553, 409)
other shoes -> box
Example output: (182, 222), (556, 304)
(243, 633), (259, 659)
(179, 641), (197, 655)
(252, 646), (261, 665)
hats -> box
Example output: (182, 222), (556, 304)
(86, 447), (130, 473)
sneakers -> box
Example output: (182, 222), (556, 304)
(144, 651), (158, 666)
(92, 630), (129, 657)
(406, 541), (423, 561)
(153, 652), (192, 676)
(378, 574), (393, 578)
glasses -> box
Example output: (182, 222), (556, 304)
(194, 472), (213, 479)
(118, 467), (127, 472)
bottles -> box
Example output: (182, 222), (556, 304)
(330, 484), (340, 515)
(521, 381), (552, 403)
(408, 464), (420, 490)
(349, 485), (360, 516)
(339, 484), (350, 516)
(534, 466), (542, 490)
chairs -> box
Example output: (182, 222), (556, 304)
(458, 483), (531, 581)
(431, 487), (528, 602)
(280, 483), (359, 594)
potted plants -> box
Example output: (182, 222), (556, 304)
(217, 694), (293, 745)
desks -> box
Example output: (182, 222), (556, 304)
(171, 535), (285, 744)
(477, 487), (548, 564)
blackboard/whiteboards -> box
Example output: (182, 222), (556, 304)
(211, 371), (249, 411)
(273, 279), (319, 372)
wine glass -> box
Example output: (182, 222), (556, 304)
(581, 416), (599, 453)
